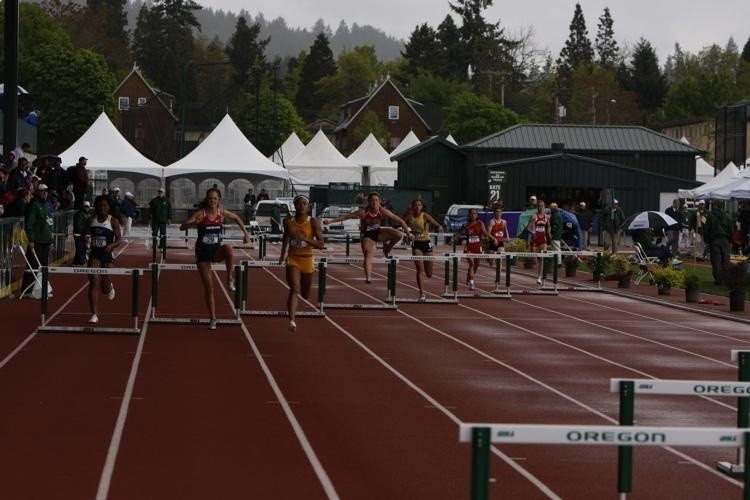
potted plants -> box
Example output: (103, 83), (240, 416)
(685, 274), (700, 301)
(587, 249), (612, 282)
(613, 254), (632, 288)
(649, 268), (684, 295)
(729, 261), (746, 312)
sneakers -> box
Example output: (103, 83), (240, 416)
(365, 278), (372, 284)
(208, 319), (217, 330)
(420, 295), (425, 301)
(469, 283), (474, 290)
(536, 278), (543, 286)
(89, 314), (99, 323)
(383, 243), (390, 257)
(107, 282), (115, 300)
(289, 320), (296, 331)
(228, 279), (236, 291)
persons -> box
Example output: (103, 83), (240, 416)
(527, 196), (537, 210)
(575, 202), (591, 251)
(664, 199), (748, 285)
(179, 183), (269, 332)
(0, 138), (172, 324)
(487, 205), (510, 272)
(528, 200), (550, 287)
(322, 191), (418, 283)
(455, 207), (490, 291)
(277, 195), (325, 331)
(631, 225), (683, 267)
(548, 203), (562, 268)
(611, 199), (624, 242)
(393, 197), (444, 303)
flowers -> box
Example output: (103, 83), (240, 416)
(563, 254), (587, 265)
(505, 238), (528, 270)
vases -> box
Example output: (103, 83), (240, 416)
(566, 266), (576, 277)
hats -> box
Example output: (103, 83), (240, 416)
(29, 157), (133, 208)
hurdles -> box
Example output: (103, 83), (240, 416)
(36, 265), (143, 335)
(441, 253), (514, 300)
(320, 257), (398, 310)
(240, 259), (326, 317)
(537, 249), (605, 292)
(459, 349), (750, 500)
(385, 254), (460, 305)
(492, 251), (561, 296)
(146, 261), (243, 326)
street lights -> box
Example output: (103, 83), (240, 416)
(605, 98), (617, 124)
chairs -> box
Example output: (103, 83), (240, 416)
(633, 242), (661, 286)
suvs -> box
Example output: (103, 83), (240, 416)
(442, 204), (486, 243)
(249, 199), (292, 235)
(320, 207), (360, 243)
